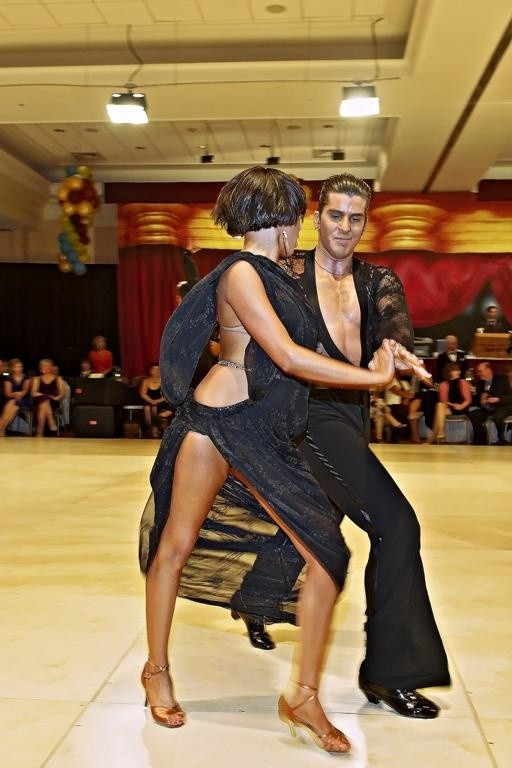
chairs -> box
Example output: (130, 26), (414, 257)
(370, 402), (512, 444)
(0, 378), (170, 437)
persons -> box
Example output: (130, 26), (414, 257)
(482, 305), (511, 333)
(88, 336), (113, 376)
(232, 174), (452, 719)
(0, 358), (66, 436)
(140, 362), (165, 436)
(369, 334), (474, 445)
(469, 362), (512, 445)
(139, 166), (394, 752)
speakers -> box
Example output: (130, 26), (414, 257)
(72, 404), (115, 436)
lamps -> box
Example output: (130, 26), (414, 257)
(338, 16), (388, 118)
(107, 23), (151, 126)
(266, 142), (281, 166)
(201, 143), (216, 164)
(332, 138), (345, 161)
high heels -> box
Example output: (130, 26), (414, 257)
(228, 590), (276, 653)
(356, 661), (442, 722)
(138, 658), (187, 732)
(275, 675), (353, 758)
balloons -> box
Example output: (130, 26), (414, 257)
(54, 165), (105, 277)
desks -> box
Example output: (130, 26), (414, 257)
(418, 355), (512, 381)
